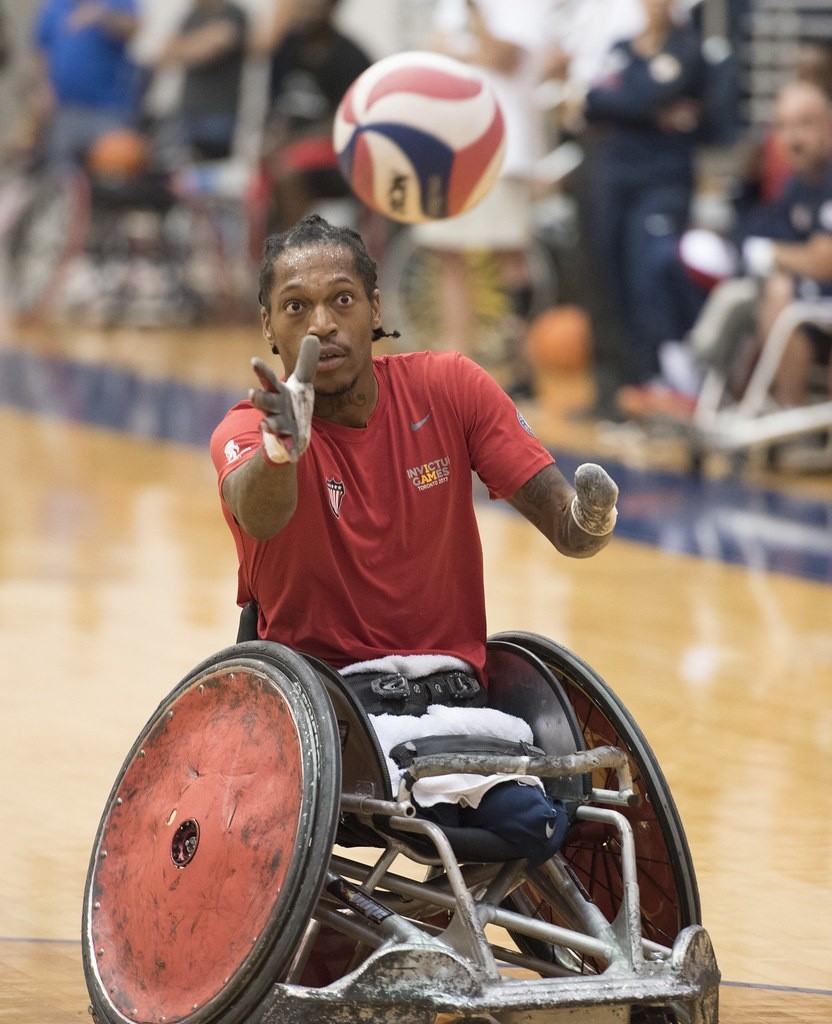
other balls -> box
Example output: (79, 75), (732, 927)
(332, 46), (505, 221)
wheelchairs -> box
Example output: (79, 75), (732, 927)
(82, 599), (721, 1024)
(1, 143), (831, 485)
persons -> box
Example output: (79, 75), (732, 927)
(1, 0), (831, 416)
(208, 210), (623, 866)
